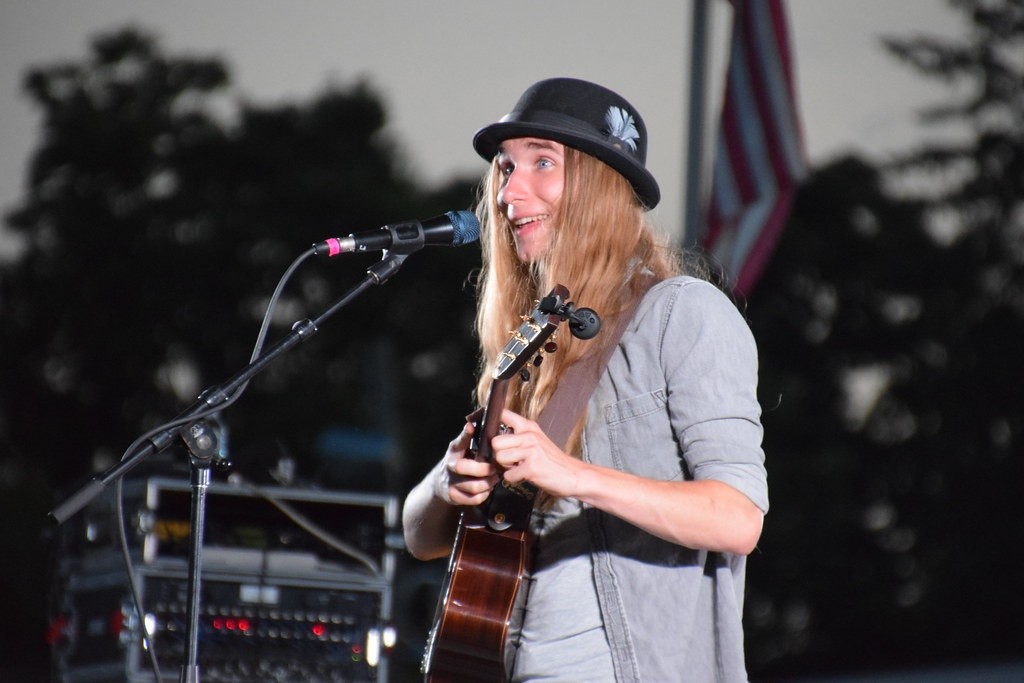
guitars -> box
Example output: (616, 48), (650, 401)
(419, 279), (603, 683)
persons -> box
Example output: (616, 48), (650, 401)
(401, 79), (769, 683)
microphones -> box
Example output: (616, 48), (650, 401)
(312, 209), (481, 256)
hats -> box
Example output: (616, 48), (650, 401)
(470, 78), (660, 213)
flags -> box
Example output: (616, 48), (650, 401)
(703, 0), (804, 301)
